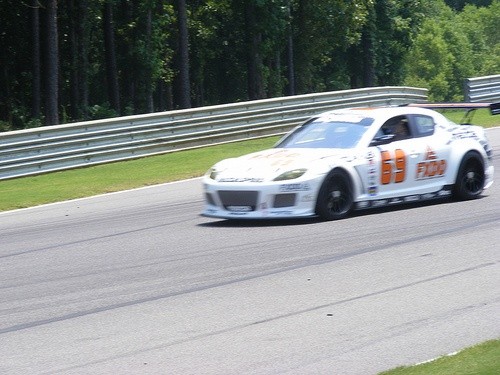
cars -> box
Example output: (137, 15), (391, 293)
(201, 101), (500, 221)
